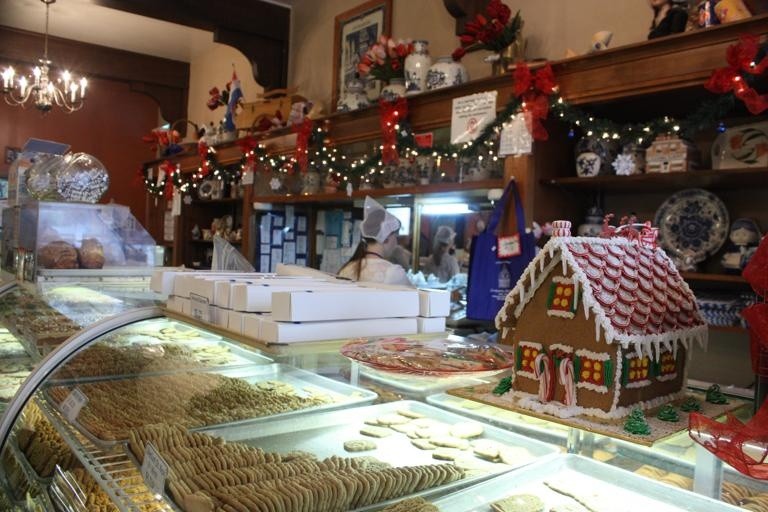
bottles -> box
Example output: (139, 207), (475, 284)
(575, 217), (604, 236)
(210, 169), (245, 200)
(405, 37), (430, 95)
(199, 120), (240, 147)
(297, 152), (486, 194)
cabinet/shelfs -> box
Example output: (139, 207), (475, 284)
(248, 73), (531, 287)
(532, 17), (766, 333)
(145, 138), (248, 270)
(0, 276), (768, 511)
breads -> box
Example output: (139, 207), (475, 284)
(38, 238), (106, 269)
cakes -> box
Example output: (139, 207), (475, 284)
(495, 212), (709, 425)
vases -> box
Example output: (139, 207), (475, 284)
(380, 78), (406, 97)
(403, 40), (433, 93)
(490, 33), (526, 76)
(426, 56), (470, 91)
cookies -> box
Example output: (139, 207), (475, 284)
(0, 286), (542, 512)
(458, 399), (768, 511)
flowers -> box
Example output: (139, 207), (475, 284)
(451, 1), (526, 63)
(353, 36), (415, 81)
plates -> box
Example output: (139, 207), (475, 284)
(649, 189), (730, 262)
(339, 335), (513, 379)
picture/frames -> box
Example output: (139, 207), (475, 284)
(331, 0), (393, 112)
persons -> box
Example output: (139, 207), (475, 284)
(337, 210), (465, 301)
(424, 226), (461, 283)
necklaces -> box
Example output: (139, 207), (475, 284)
(364, 252), (384, 258)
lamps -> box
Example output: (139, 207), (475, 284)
(0, 0), (88, 117)
